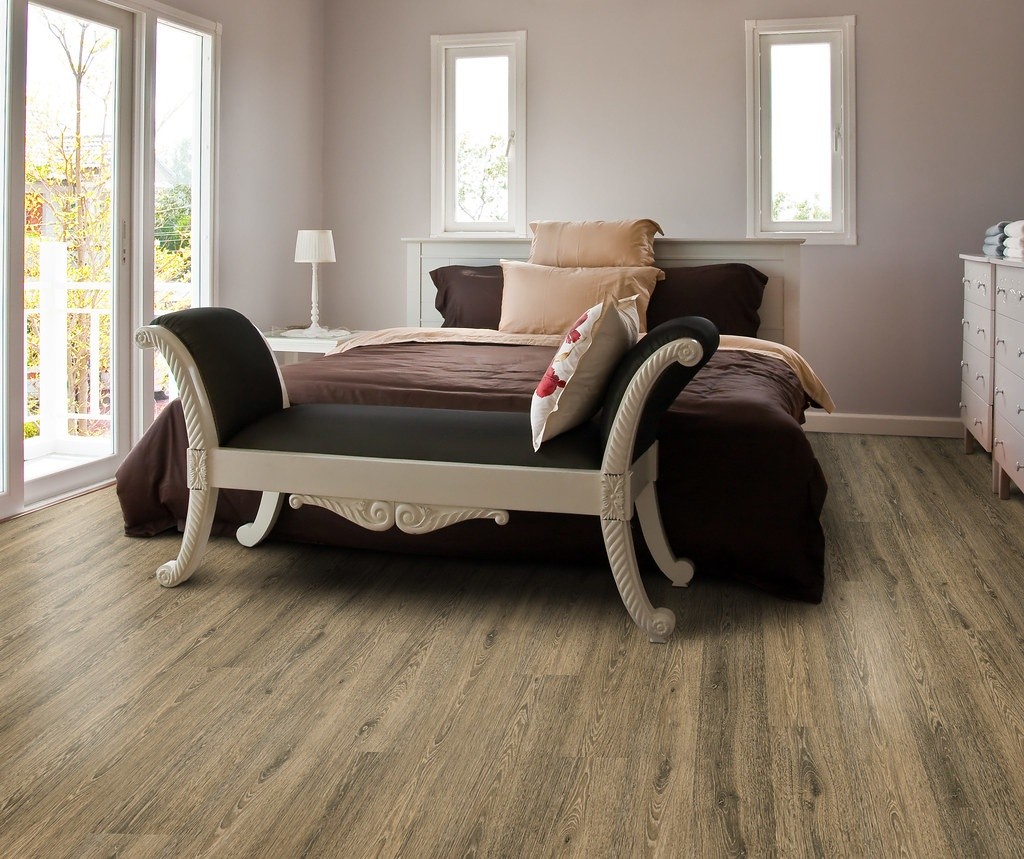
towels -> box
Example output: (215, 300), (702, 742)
(983, 220), (1024, 257)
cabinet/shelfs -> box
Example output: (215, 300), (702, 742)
(958, 252), (1024, 499)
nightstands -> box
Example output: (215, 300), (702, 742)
(263, 326), (370, 366)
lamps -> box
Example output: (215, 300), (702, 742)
(281, 230), (351, 338)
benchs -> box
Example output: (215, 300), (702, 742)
(134, 306), (719, 644)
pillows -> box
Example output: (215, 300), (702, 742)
(527, 219), (664, 269)
(531, 290), (643, 451)
(645, 263), (769, 339)
(499, 258), (665, 335)
(428, 265), (503, 331)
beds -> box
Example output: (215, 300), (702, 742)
(115, 218), (834, 606)
